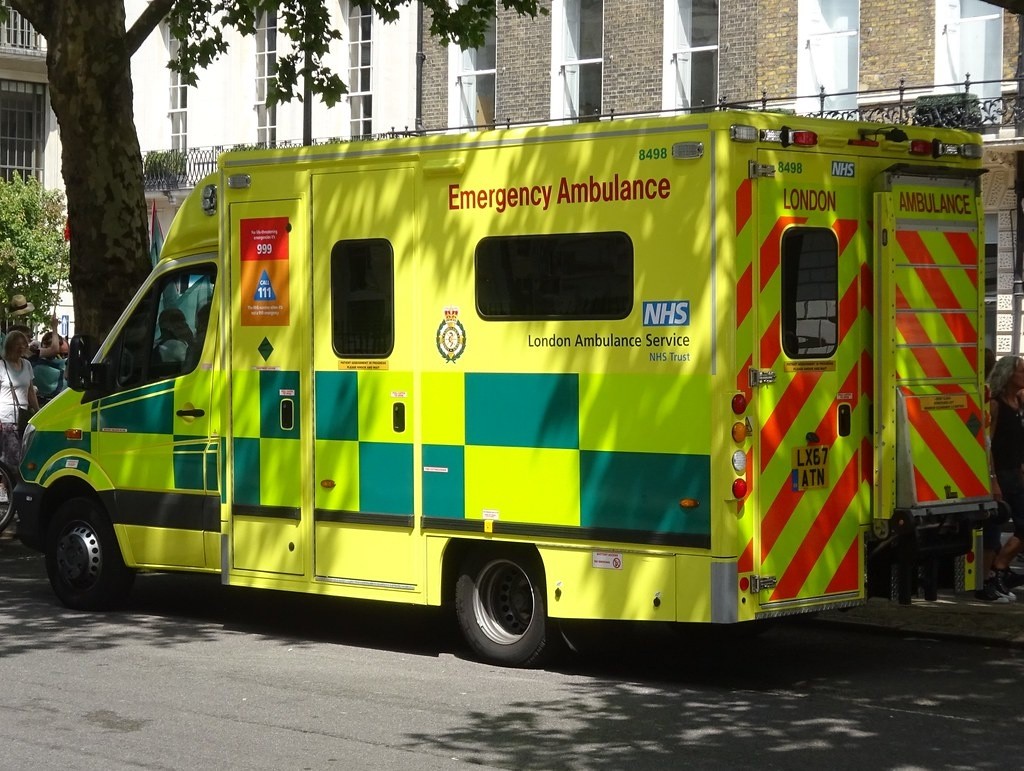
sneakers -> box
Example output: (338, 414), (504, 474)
(972, 568), (1016, 604)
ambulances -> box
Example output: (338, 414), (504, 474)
(16, 110), (1022, 670)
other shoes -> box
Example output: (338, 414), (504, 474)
(1001, 566), (1024, 592)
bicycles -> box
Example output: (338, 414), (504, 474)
(0, 463), (15, 534)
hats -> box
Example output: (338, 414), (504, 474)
(8, 294), (35, 318)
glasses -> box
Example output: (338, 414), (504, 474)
(46, 339), (63, 347)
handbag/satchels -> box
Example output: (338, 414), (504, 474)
(17, 407), (39, 443)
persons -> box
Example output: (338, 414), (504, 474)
(138, 308), (199, 384)
(6, 325), (70, 403)
(983, 354), (1024, 604)
(0, 331), (40, 502)
